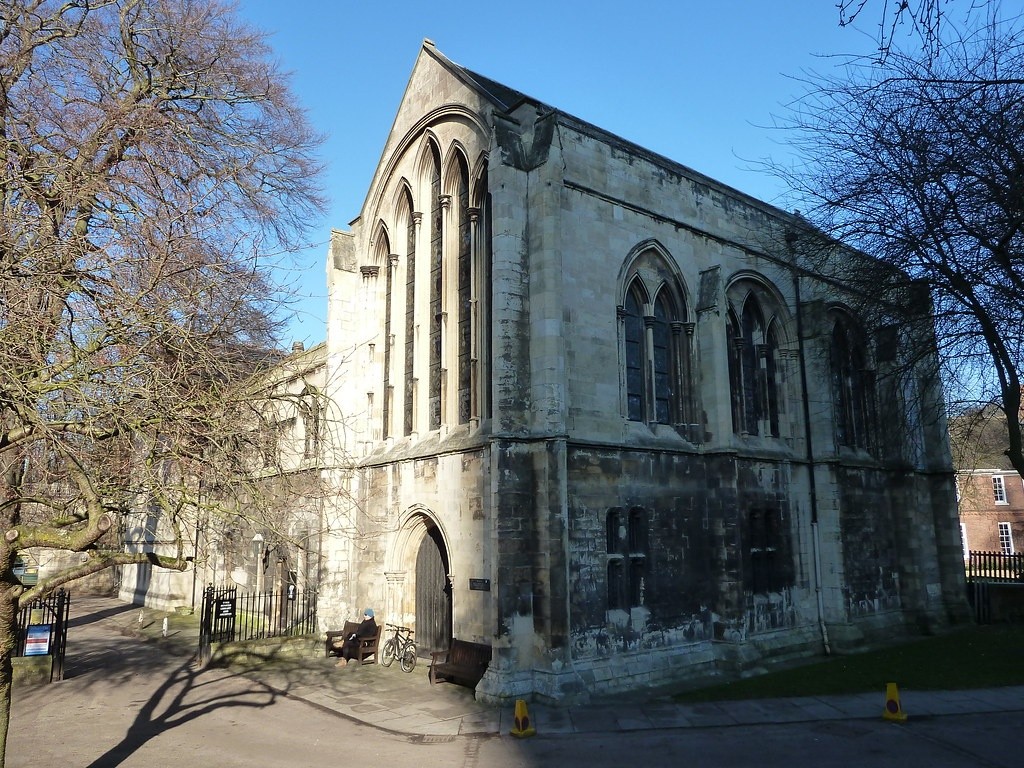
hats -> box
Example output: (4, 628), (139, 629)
(364, 609), (374, 617)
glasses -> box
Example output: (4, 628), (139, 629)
(365, 614), (368, 617)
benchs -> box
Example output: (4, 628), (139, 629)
(326, 618), (382, 665)
(429, 637), (492, 701)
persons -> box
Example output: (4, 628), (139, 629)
(335, 609), (376, 667)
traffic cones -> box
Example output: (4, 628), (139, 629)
(510, 700), (537, 737)
(882, 683), (909, 722)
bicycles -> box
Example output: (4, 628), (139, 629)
(381, 623), (417, 673)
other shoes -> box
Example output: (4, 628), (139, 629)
(335, 657), (347, 668)
(333, 640), (344, 648)
